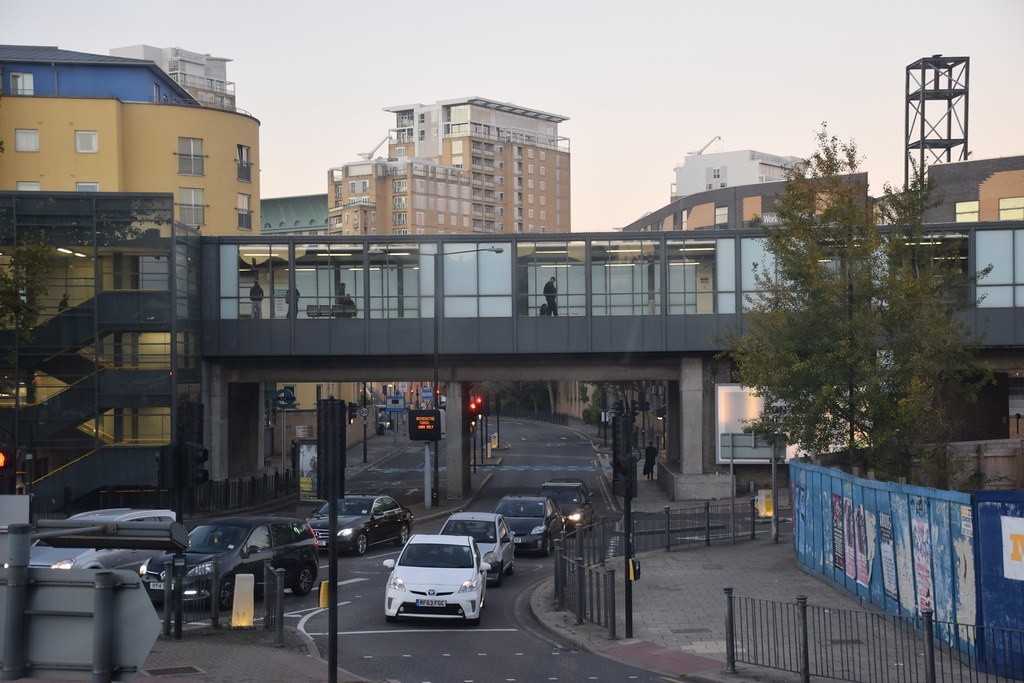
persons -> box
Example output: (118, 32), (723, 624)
(338, 282), (346, 304)
(645, 440), (657, 480)
(59, 292), (70, 309)
(285, 284), (300, 319)
(543, 277), (558, 316)
(250, 280), (264, 319)
(345, 293), (356, 317)
(540, 303), (550, 316)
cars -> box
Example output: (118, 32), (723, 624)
(429, 512), (516, 586)
(304, 494), (413, 556)
(384, 534), (492, 627)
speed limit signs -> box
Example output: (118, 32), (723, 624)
(360, 408), (370, 418)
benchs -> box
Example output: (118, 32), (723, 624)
(307, 303), (358, 319)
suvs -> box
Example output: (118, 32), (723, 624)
(140, 516), (319, 613)
(492, 495), (562, 557)
(536, 482), (595, 534)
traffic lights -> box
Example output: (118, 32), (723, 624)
(410, 389), (414, 394)
(644, 401), (650, 412)
(348, 402), (357, 424)
(437, 390), (440, 394)
(630, 558), (641, 581)
(0, 450), (9, 470)
(477, 396), (483, 414)
(186, 443), (211, 489)
(471, 402), (477, 421)
(631, 399), (641, 424)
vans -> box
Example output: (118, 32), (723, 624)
(27, 508), (177, 602)
